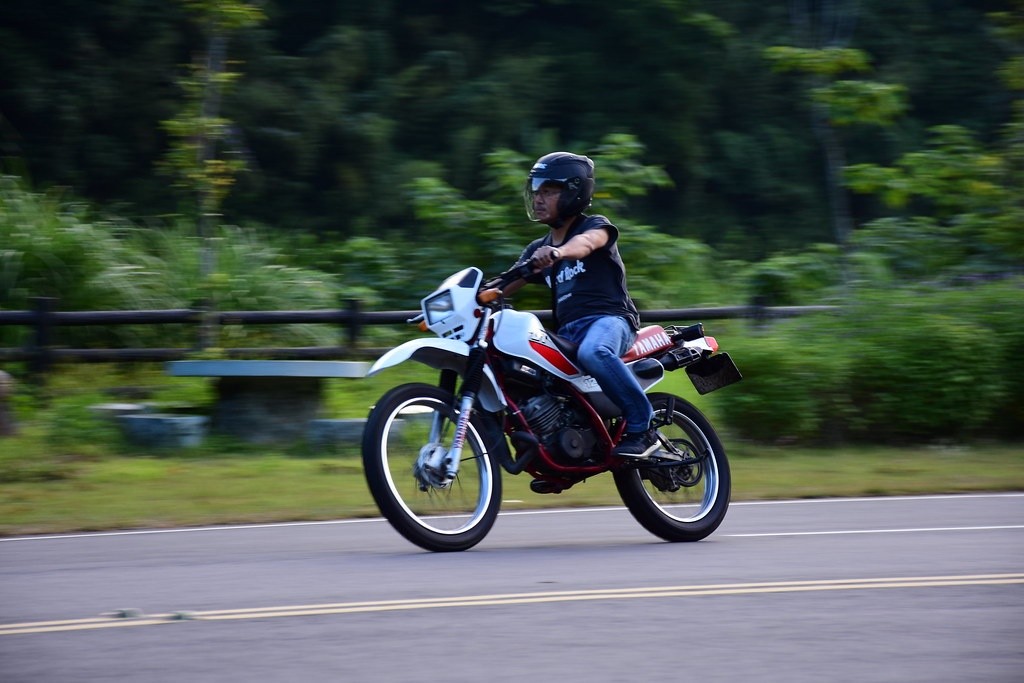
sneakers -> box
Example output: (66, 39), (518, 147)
(530, 478), (555, 493)
(612, 421), (662, 457)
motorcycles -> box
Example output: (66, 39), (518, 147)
(361, 249), (743, 551)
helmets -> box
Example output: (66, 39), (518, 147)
(529, 152), (595, 219)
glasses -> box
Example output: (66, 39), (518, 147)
(531, 190), (560, 197)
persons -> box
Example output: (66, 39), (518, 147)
(475, 150), (666, 495)
